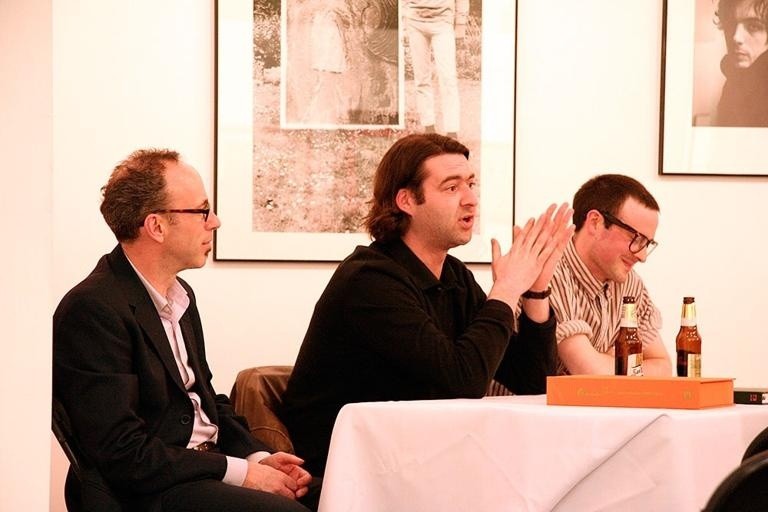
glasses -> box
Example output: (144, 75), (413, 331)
(584, 206), (658, 257)
(140, 201), (211, 227)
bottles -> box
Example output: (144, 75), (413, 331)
(613, 296), (645, 377)
(674, 296), (703, 381)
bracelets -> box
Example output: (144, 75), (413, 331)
(519, 287), (553, 300)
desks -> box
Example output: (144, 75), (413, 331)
(316, 396), (768, 512)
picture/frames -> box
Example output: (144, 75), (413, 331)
(658, 0), (768, 178)
(213, 0), (520, 267)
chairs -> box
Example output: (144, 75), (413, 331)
(52, 416), (84, 483)
(232, 363), (294, 453)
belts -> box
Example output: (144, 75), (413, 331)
(192, 441), (217, 452)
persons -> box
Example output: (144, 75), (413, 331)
(482, 172), (674, 397)
(52, 148), (324, 512)
(711, 1), (768, 127)
(280, 130), (578, 477)
(402, 1), (470, 139)
